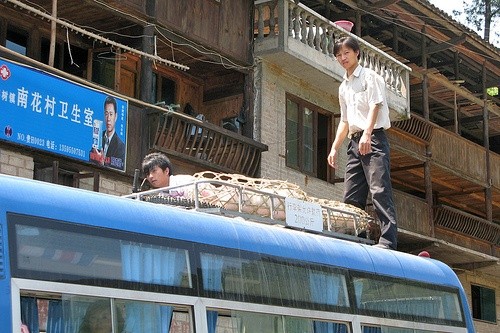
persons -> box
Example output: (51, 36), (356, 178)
(327, 38), (397, 251)
(141, 152), (382, 246)
(87, 96), (124, 169)
(78, 298), (124, 333)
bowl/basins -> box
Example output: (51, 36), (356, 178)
(334, 20), (354, 32)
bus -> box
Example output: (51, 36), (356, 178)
(0, 169), (477, 333)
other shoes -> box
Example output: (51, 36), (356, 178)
(373, 244), (394, 250)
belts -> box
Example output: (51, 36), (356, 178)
(351, 128), (384, 139)
